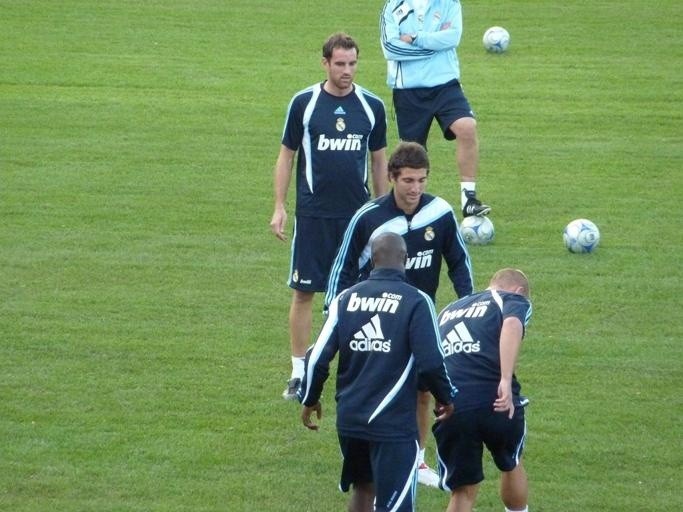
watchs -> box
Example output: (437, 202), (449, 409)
(410, 31), (418, 44)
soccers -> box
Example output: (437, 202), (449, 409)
(481, 25), (511, 54)
(459, 214), (494, 245)
(564, 218), (599, 253)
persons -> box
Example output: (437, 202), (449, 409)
(415, 268), (533, 512)
(379, 0), (492, 216)
(320, 142), (474, 489)
(296, 231), (459, 512)
(270, 34), (391, 401)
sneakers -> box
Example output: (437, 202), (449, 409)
(283, 377), (302, 399)
(462, 191), (492, 217)
(417, 463), (439, 488)
